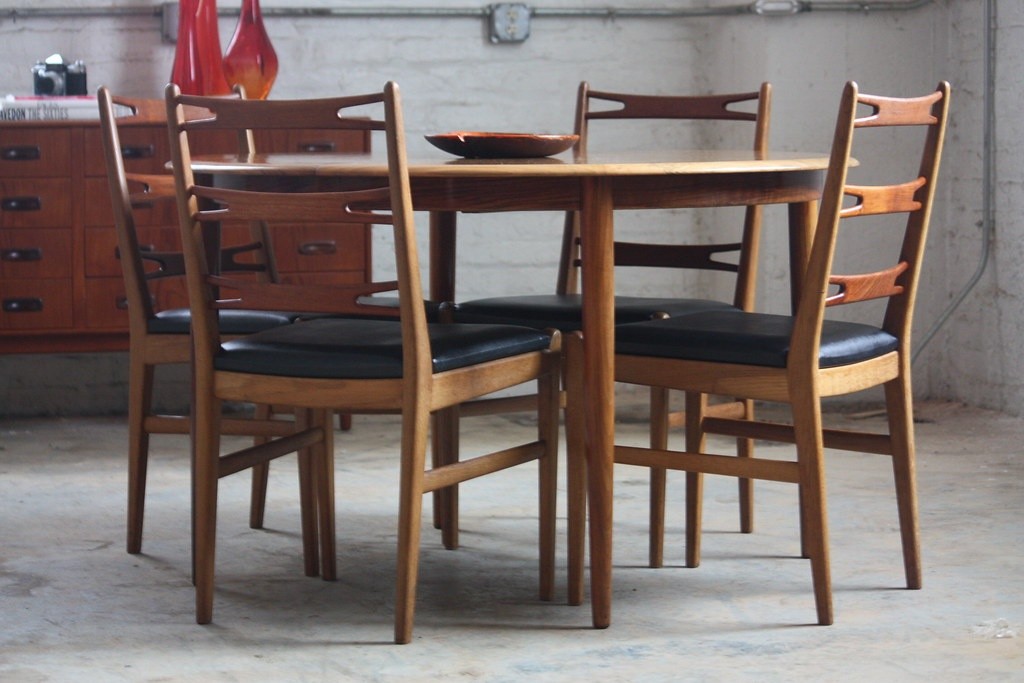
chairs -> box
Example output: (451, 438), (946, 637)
(443, 79), (776, 572)
(566, 79), (953, 622)
(97, 86), (446, 584)
(165, 78), (568, 647)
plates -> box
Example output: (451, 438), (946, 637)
(426, 127), (579, 157)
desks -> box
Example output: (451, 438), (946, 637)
(163, 155), (860, 632)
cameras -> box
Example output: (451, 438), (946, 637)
(32, 54), (88, 97)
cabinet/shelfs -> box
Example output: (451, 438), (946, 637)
(0, 95), (373, 433)
